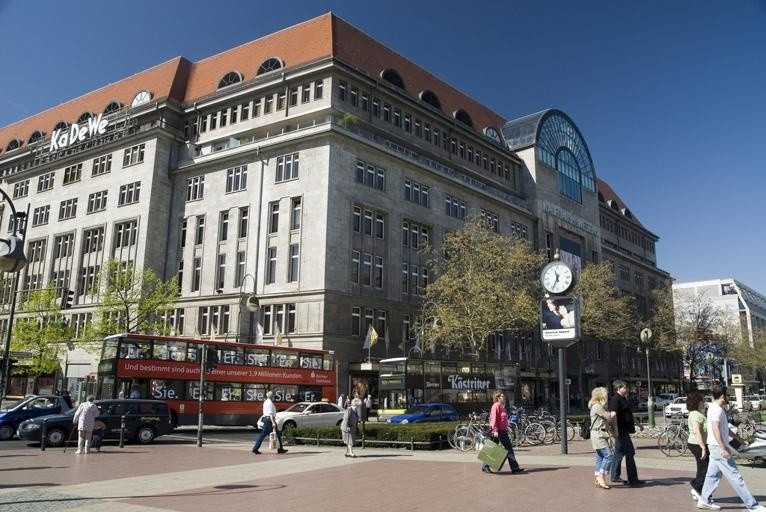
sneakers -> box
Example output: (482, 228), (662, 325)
(748, 505), (766, 511)
(252, 448), (261, 454)
(691, 489), (721, 510)
(344, 452), (356, 458)
(278, 448), (288, 453)
(75, 449), (90, 454)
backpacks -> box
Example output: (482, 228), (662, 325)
(580, 414), (600, 439)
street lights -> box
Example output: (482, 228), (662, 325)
(534, 356), (551, 405)
(233, 273), (262, 343)
(0, 212), (35, 412)
(420, 299), (442, 357)
(639, 328), (658, 428)
(705, 352), (716, 391)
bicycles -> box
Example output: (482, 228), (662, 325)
(657, 403), (761, 459)
(446, 404), (575, 452)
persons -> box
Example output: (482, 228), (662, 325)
(686, 392), (707, 500)
(611, 379), (645, 487)
(556, 304), (575, 327)
(72, 396), (100, 455)
(481, 390), (525, 473)
(697, 386), (766, 512)
(251, 392), (288, 454)
(587, 385), (617, 491)
(542, 300), (562, 328)
(338, 392), (373, 458)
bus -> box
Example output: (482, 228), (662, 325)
(96, 333), (341, 427)
(378, 353), (523, 419)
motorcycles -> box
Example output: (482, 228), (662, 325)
(729, 424), (766, 467)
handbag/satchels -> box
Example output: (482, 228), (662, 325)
(269, 428), (280, 450)
(476, 439), (509, 471)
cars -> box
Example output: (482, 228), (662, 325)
(254, 399), (354, 432)
(387, 403), (460, 424)
(635, 392), (765, 419)
(0, 393), (73, 439)
(15, 397), (172, 446)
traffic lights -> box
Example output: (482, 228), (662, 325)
(57, 285), (74, 311)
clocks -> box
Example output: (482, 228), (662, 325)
(540, 247), (576, 295)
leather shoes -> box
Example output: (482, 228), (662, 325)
(482, 467), (492, 474)
(511, 468), (523, 473)
(595, 475), (645, 489)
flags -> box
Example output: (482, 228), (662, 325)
(362, 325), (379, 349)
